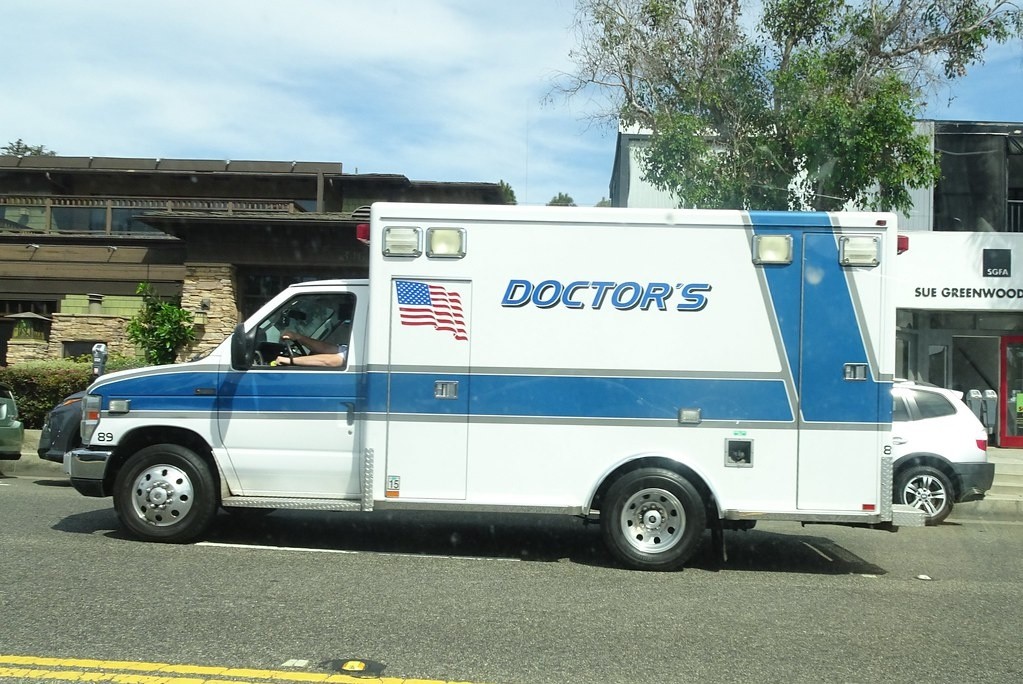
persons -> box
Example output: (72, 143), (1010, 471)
(275, 331), (348, 368)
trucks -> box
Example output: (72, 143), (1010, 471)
(37, 201), (909, 571)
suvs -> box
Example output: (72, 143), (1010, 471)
(893, 377), (995, 525)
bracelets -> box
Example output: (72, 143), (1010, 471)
(289, 356), (294, 365)
